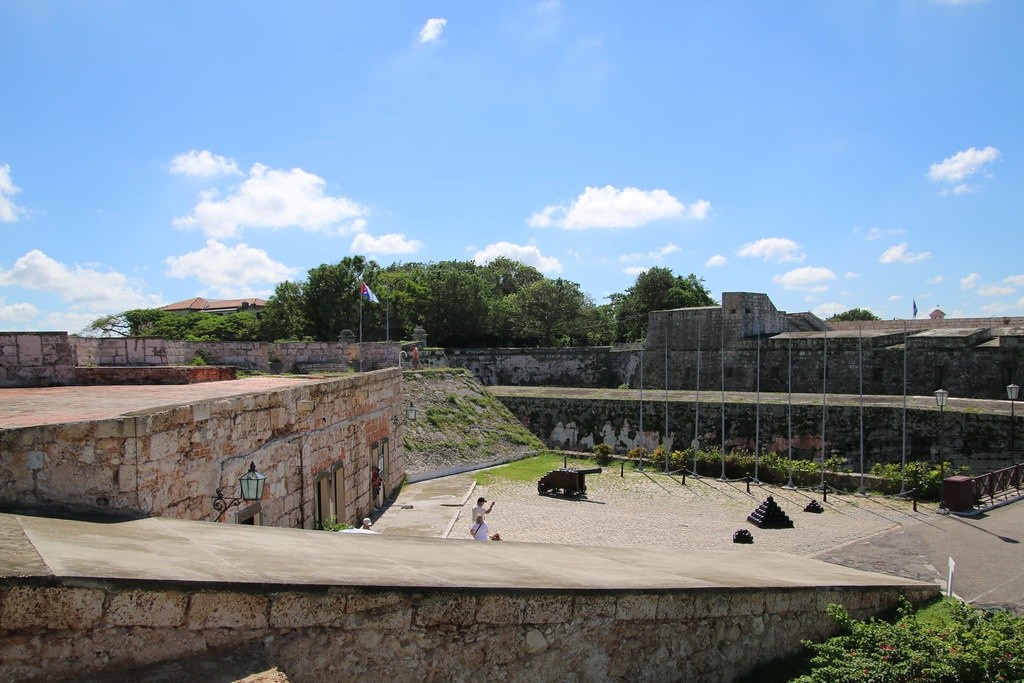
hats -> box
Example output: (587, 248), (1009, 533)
(477, 497), (487, 502)
(363, 518), (372, 526)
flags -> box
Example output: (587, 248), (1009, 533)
(913, 300), (918, 318)
(360, 282), (380, 304)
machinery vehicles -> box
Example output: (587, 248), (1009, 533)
(537, 468), (602, 497)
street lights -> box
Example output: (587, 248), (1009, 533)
(934, 385), (949, 509)
(1006, 381), (1020, 466)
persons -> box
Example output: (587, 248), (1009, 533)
(412, 344), (423, 371)
(472, 497), (495, 524)
(372, 466), (384, 502)
(470, 514), (489, 540)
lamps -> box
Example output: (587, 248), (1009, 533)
(391, 402), (418, 432)
(212, 461), (268, 522)
